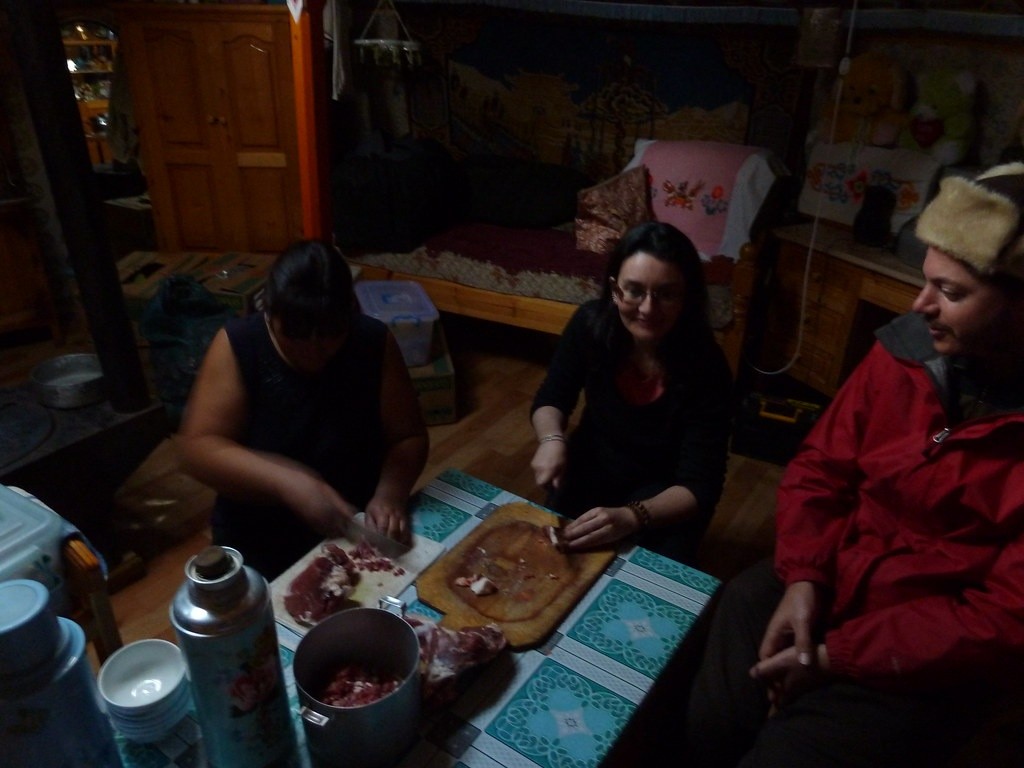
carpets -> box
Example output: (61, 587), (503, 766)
(729, 367), (837, 467)
(115, 250), (278, 314)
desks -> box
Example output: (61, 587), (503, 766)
(113, 466), (722, 768)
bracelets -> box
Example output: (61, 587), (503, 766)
(626, 501), (653, 531)
(536, 434), (569, 444)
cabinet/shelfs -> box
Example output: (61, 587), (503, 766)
(58, 32), (119, 166)
(772, 220), (929, 402)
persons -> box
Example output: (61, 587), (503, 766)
(524, 222), (739, 564)
(657, 161), (1024, 768)
(171, 238), (429, 583)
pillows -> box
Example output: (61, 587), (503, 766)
(571, 165), (655, 280)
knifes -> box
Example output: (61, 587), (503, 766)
(329, 507), (410, 558)
(547, 481), (557, 527)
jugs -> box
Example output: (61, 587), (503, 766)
(0, 580), (124, 768)
(168, 546), (294, 768)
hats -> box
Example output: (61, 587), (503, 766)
(914, 162), (1024, 283)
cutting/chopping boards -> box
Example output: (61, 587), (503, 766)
(415, 502), (615, 646)
(268, 511), (446, 636)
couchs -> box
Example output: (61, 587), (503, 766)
(330, 113), (789, 388)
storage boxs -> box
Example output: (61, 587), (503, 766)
(355, 277), (463, 427)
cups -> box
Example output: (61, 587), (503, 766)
(294, 596), (421, 768)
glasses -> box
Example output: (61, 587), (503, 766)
(615, 281), (681, 305)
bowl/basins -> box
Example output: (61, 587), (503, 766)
(95, 638), (192, 742)
(31, 354), (103, 407)
